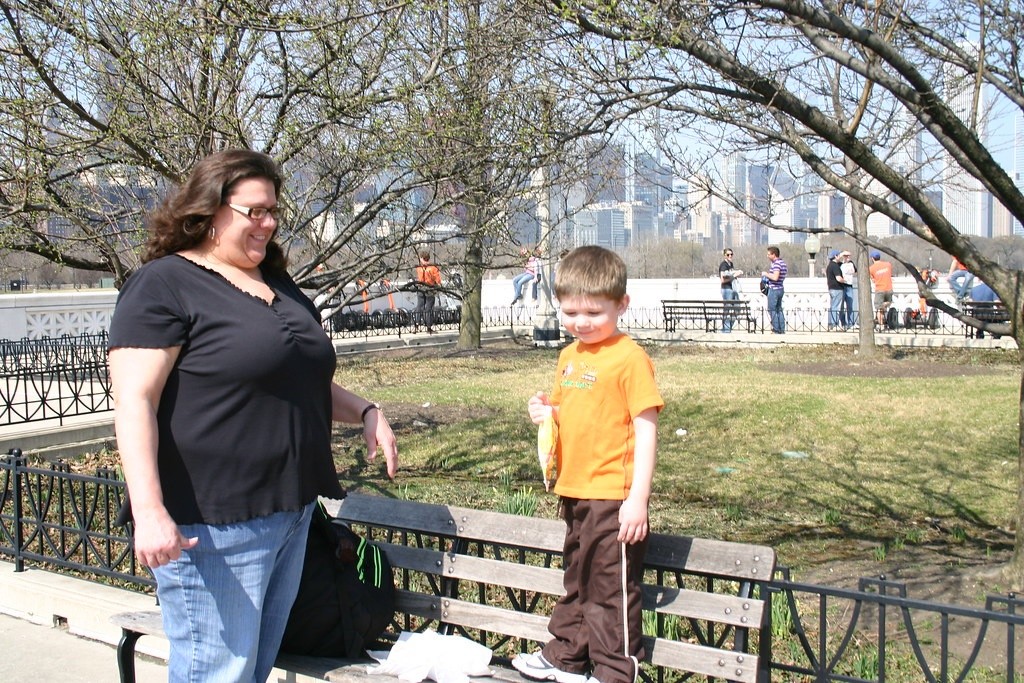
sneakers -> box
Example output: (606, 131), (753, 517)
(512, 650), (602, 683)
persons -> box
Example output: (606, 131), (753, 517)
(412, 251), (441, 335)
(554, 249), (570, 273)
(511, 256), (535, 304)
(532, 258), (541, 302)
(825, 251), (1006, 340)
(718, 248), (744, 333)
(510, 246), (664, 683)
(761, 246), (788, 334)
(108, 149), (400, 683)
(313, 263), (341, 331)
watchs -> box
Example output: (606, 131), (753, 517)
(361, 403), (382, 422)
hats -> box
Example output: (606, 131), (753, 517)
(829, 250), (841, 259)
(869, 252), (880, 259)
(842, 251), (851, 256)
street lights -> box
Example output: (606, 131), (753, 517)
(533, 79), (559, 341)
(804, 233), (821, 329)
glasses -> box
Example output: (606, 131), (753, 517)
(725, 253), (733, 256)
(224, 202), (285, 220)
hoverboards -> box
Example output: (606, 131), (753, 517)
(873, 308), (898, 329)
(904, 272), (939, 330)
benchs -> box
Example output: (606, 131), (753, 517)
(961, 301), (1011, 339)
(660, 299), (757, 334)
(109, 492), (778, 683)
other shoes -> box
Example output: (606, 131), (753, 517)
(843, 323), (859, 329)
(772, 330), (784, 334)
(977, 333), (985, 338)
(956, 298), (962, 305)
(830, 326), (843, 331)
(518, 296), (523, 299)
(510, 299), (517, 305)
(427, 329), (437, 334)
(876, 324), (885, 331)
(994, 334), (1001, 338)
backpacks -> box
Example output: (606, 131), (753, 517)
(278, 501), (397, 660)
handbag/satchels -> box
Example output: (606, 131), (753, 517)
(760, 280), (769, 296)
